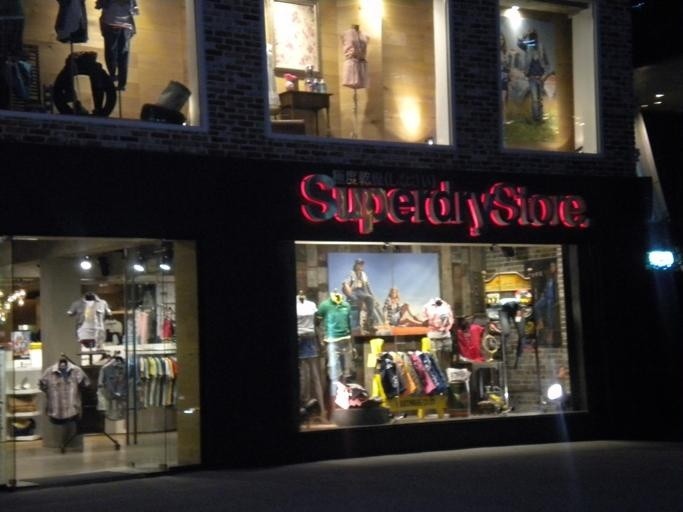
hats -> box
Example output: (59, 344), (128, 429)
(355, 258), (365, 264)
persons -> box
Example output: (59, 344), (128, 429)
(310, 294), (358, 408)
(423, 298), (455, 363)
(518, 30), (554, 123)
(341, 258), (378, 332)
(382, 287), (422, 328)
(339, 24), (371, 91)
(53, 1), (89, 44)
(296, 289), (321, 409)
(529, 261), (561, 347)
(92, 0), (138, 92)
(497, 33), (514, 128)
(65, 291), (112, 348)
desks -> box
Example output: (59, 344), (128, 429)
(278, 92), (335, 136)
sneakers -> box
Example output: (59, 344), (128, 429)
(537, 112), (550, 124)
(363, 325), (379, 332)
(502, 120), (515, 126)
(423, 319), (429, 327)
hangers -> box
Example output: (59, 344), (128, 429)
(59, 353), (67, 374)
(101, 352), (176, 363)
